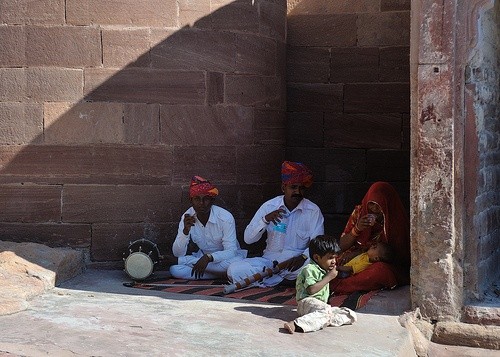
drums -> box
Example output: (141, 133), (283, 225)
(121, 236), (165, 280)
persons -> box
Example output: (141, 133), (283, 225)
(169, 176), (244, 280)
(329, 182), (409, 296)
(283, 234), (357, 335)
(337, 247), (392, 278)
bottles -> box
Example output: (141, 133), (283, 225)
(268, 208), (290, 252)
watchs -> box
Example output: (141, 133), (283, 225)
(300, 254), (308, 260)
(227, 161), (325, 288)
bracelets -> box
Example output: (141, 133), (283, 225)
(206, 253), (213, 262)
(352, 223), (361, 236)
